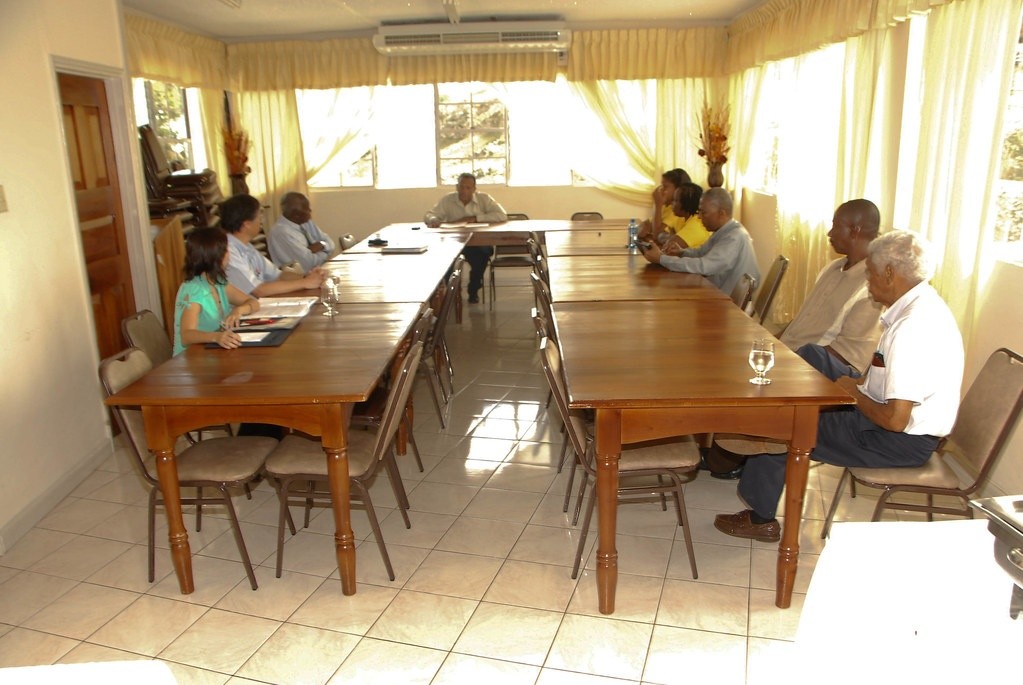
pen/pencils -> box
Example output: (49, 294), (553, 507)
(217, 322), (228, 330)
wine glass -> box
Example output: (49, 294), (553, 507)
(321, 284), (340, 317)
(748, 339), (775, 385)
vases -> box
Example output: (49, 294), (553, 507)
(230, 174), (249, 197)
(707, 163), (724, 187)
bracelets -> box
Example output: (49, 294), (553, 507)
(247, 302), (252, 315)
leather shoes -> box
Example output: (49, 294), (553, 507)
(713, 510), (781, 542)
(696, 447), (746, 479)
(713, 433), (791, 455)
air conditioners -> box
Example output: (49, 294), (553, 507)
(373, 20), (572, 57)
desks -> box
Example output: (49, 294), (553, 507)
(106, 219), (857, 615)
(797, 520), (1023, 683)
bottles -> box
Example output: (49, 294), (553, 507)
(627, 219), (639, 254)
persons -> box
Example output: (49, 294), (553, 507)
(220, 194), (330, 299)
(697, 199), (887, 480)
(714, 230), (964, 543)
(642, 187), (762, 307)
(423, 173), (507, 303)
(657, 183), (713, 250)
(266, 192), (335, 276)
(637, 168), (691, 239)
(172, 228), (283, 482)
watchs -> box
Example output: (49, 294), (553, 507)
(320, 240), (326, 246)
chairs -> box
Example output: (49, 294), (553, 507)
(98, 123), (1023, 591)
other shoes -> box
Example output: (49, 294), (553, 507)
(468, 288), (479, 303)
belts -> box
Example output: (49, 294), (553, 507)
(823, 345), (860, 371)
(924, 435), (943, 441)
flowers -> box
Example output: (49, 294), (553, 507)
(219, 122), (251, 174)
(691, 94), (730, 162)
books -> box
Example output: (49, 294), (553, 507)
(382, 244), (428, 253)
